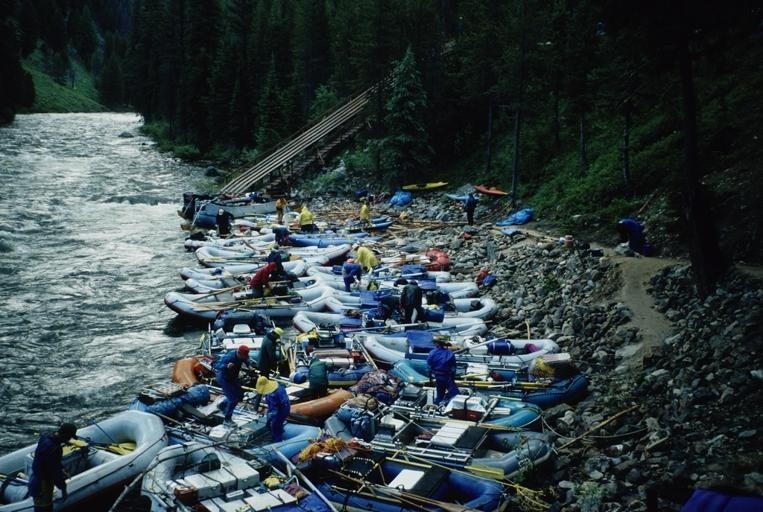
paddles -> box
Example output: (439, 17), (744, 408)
(373, 486), (481, 511)
(333, 323), (419, 336)
(34, 432), (137, 456)
(190, 254), (301, 312)
(453, 338), (498, 354)
(424, 458), (505, 480)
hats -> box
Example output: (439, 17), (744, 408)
(256, 377), (279, 395)
(239, 345), (249, 361)
(219, 209), (224, 215)
(272, 327), (284, 336)
(61, 423), (79, 440)
(432, 335), (453, 346)
(351, 243), (360, 252)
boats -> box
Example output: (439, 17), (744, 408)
(0, 403), (168, 512)
(129, 192), (592, 512)
(472, 184), (508, 196)
(139, 439), (331, 512)
(401, 180), (449, 192)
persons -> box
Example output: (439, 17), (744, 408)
(351, 242), (380, 271)
(275, 197), (286, 224)
(273, 226), (292, 244)
(463, 191), (476, 223)
(248, 262), (276, 297)
(358, 200), (371, 232)
(214, 344), (254, 424)
(298, 207), (315, 234)
(252, 375), (290, 442)
(399, 278), (426, 322)
(269, 243), (291, 262)
(426, 332), (460, 398)
(215, 209), (234, 235)
(341, 258), (361, 292)
(252, 324), (283, 413)
(25, 421), (77, 511)
(306, 356), (328, 399)
